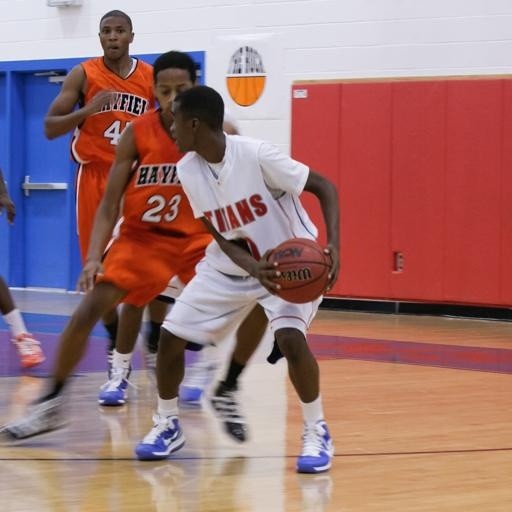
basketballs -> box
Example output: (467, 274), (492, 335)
(266, 238), (332, 303)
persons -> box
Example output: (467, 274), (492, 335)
(43, 9), (155, 386)
(134, 84), (341, 474)
(0, 168), (47, 369)
(0, 50), (269, 450)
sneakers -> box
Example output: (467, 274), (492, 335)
(296, 421), (335, 473)
(181, 358), (218, 404)
(5, 398), (71, 440)
(209, 380), (249, 440)
(98, 346), (132, 405)
(142, 341), (158, 376)
(11, 331), (46, 368)
(135, 414), (185, 460)
